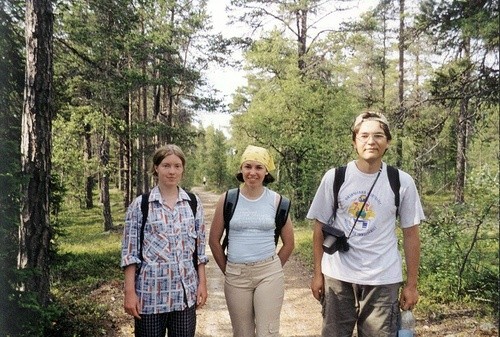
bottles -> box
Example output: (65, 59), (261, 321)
(397, 309), (415, 337)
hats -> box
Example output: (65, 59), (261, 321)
(240, 144), (275, 173)
(355, 111), (389, 128)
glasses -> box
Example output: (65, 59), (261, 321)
(357, 132), (387, 142)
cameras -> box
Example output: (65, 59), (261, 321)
(322, 225), (350, 256)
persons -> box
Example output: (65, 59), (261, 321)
(209, 145), (295, 337)
(305, 111), (426, 337)
(203, 177), (206, 186)
(120, 144), (209, 337)
